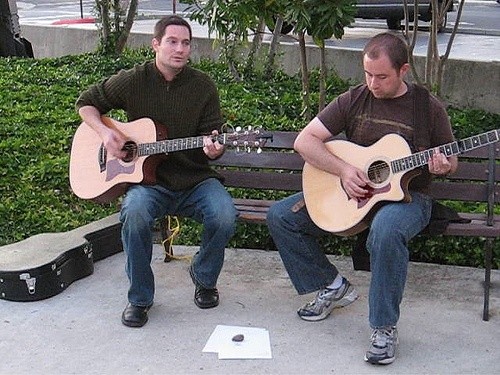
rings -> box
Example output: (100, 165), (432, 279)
(434, 168), (441, 172)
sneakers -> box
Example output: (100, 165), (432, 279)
(297, 277), (358, 321)
(365, 327), (400, 365)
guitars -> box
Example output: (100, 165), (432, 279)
(301, 127), (500, 236)
(69, 116), (274, 203)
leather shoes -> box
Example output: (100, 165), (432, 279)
(121, 300), (154, 327)
(188, 263), (220, 308)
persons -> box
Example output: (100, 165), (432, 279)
(266, 31), (458, 364)
(75, 16), (236, 327)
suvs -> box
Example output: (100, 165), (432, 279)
(268, 0), (459, 38)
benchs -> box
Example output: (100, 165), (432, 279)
(156, 132), (500, 322)
